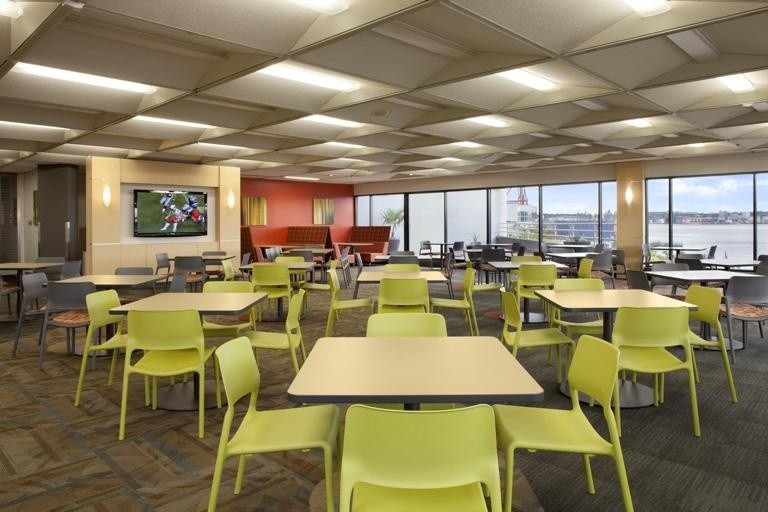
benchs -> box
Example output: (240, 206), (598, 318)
(240, 225), (393, 269)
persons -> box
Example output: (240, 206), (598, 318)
(158, 191), (206, 235)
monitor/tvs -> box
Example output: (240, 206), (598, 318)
(134, 189), (208, 238)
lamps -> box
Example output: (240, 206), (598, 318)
(103, 183), (110, 207)
(228, 192), (234, 209)
